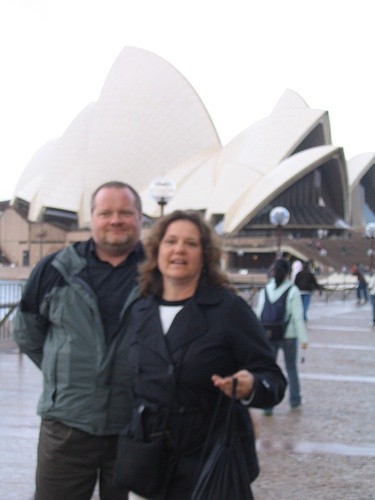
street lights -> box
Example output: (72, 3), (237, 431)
(150, 176), (175, 219)
(270, 206), (291, 260)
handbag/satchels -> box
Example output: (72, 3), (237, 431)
(193, 378), (253, 500)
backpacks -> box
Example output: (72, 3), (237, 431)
(261, 283), (296, 339)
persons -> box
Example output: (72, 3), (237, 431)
(254, 226), (375, 417)
(113, 209), (288, 500)
(11, 181), (149, 500)
